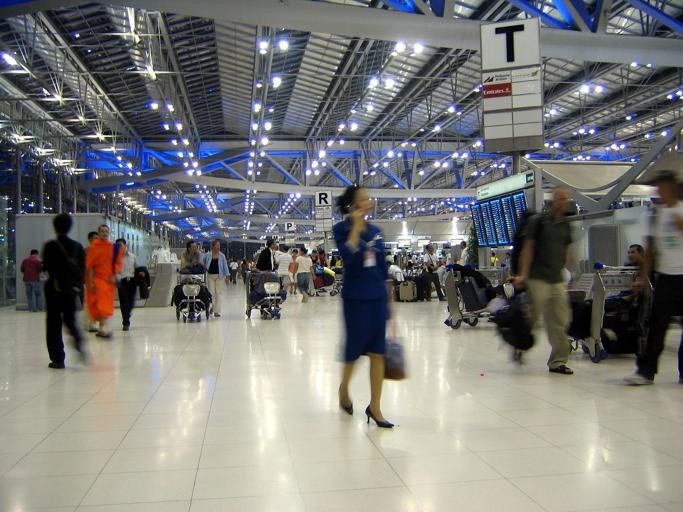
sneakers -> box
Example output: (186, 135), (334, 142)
(623, 371), (656, 385)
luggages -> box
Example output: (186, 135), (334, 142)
(399, 281), (417, 302)
(456, 276), (487, 310)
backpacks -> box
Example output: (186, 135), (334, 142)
(51, 240), (83, 292)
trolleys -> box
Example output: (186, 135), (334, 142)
(246, 269), (285, 318)
(565, 258), (650, 364)
(328, 276), (344, 296)
(439, 259), (481, 328)
(302, 264), (338, 297)
(174, 262), (214, 322)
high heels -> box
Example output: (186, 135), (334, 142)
(338, 385), (354, 416)
(365, 404), (394, 428)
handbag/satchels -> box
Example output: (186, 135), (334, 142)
(490, 279), (535, 351)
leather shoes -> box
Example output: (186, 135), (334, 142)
(549, 365), (573, 374)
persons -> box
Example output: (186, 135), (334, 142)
(507, 186), (575, 373)
(621, 243), (643, 270)
(624, 170), (683, 385)
(504, 253), (512, 264)
(332, 186), (395, 429)
(21, 250), (42, 312)
(42, 214), (85, 368)
